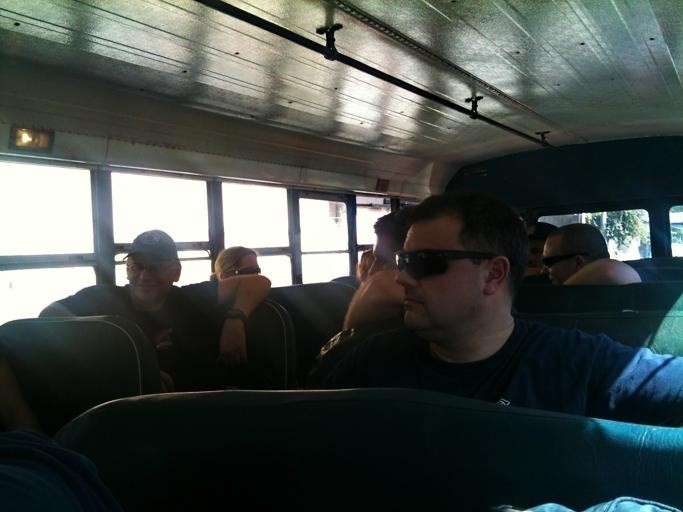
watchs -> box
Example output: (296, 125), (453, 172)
(223, 308), (251, 323)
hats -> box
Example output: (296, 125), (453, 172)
(122, 230), (178, 263)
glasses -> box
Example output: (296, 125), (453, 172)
(127, 262), (169, 276)
(234, 266), (261, 275)
(539, 255), (589, 270)
(393, 250), (495, 279)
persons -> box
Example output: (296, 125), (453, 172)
(342, 207), (657, 335)
(209, 246), (261, 281)
(37, 230), (270, 393)
(311, 188), (681, 428)
